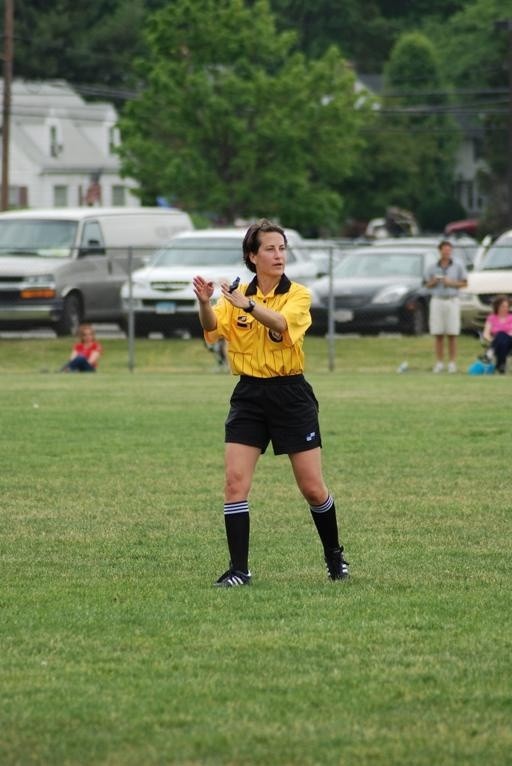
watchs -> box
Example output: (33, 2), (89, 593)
(242, 298), (256, 313)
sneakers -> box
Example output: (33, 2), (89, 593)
(478, 355), (505, 372)
(212, 569), (252, 588)
(433, 363), (457, 373)
(325, 546), (348, 583)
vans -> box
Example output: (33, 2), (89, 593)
(1, 206), (192, 334)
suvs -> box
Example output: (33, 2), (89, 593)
(118, 227), (314, 335)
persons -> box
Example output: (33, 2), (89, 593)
(194, 216), (351, 585)
(481, 291), (512, 375)
(60, 325), (102, 372)
(422, 238), (469, 374)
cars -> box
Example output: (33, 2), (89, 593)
(303, 227), (512, 330)
